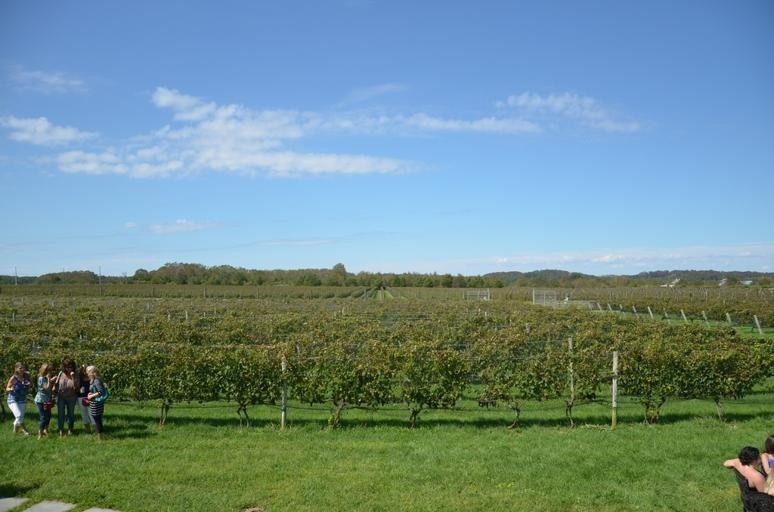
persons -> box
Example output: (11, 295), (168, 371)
(723, 434), (774, 496)
(6, 358), (105, 441)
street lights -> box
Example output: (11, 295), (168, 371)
(82, 398), (91, 406)
(43, 400), (54, 411)
(93, 383), (109, 402)
